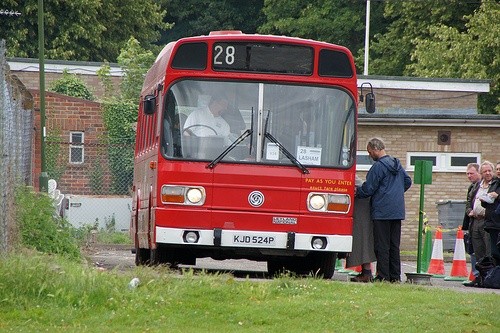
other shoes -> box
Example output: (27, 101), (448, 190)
(463, 282), (475, 287)
(370, 277), (390, 283)
(351, 273), (370, 282)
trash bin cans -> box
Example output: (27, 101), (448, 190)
(435, 199), (467, 254)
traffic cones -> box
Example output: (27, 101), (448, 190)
(348, 265), (362, 275)
(420, 231), (433, 273)
(427, 231), (446, 278)
(462, 268), (476, 285)
(338, 259), (356, 273)
(443, 230), (469, 280)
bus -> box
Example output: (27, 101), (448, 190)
(129, 29), (376, 280)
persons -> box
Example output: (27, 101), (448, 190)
(461, 161), (500, 287)
(355, 138), (412, 282)
(172, 93), (231, 137)
(345, 175), (376, 282)
